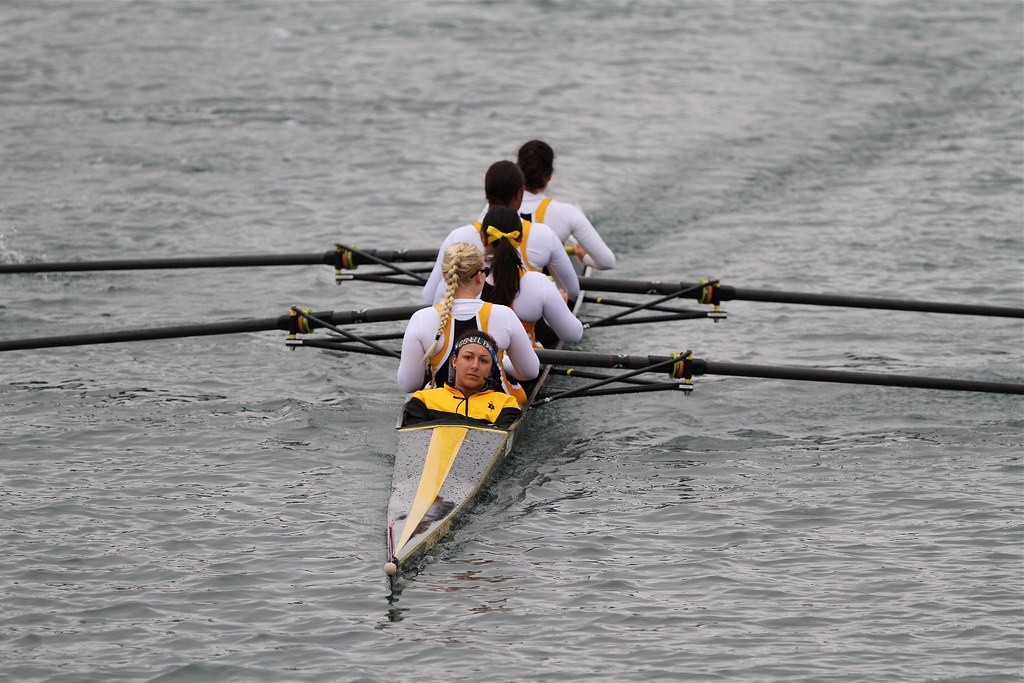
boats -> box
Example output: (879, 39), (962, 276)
(385, 234), (592, 587)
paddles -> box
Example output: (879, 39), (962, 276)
(576, 273), (1023, 321)
(2, 247), (440, 278)
(1, 306), (434, 350)
(537, 349), (1024, 393)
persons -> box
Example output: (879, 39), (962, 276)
(421, 160), (580, 302)
(479, 139), (616, 271)
(432, 206), (584, 343)
(397, 242), (540, 393)
(401, 329), (523, 430)
